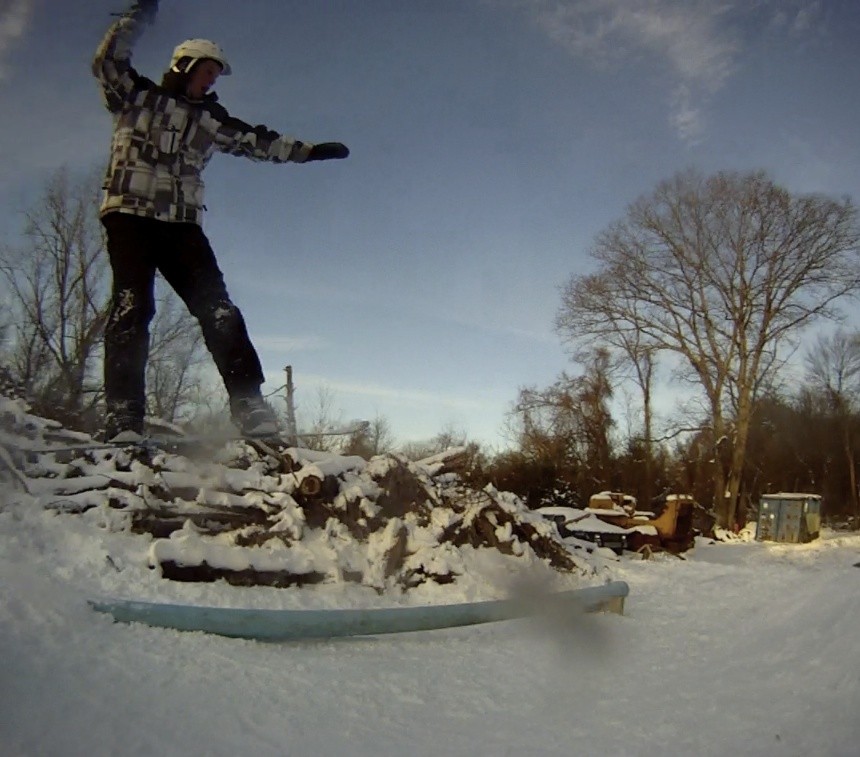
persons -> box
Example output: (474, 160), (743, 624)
(92, 0), (353, 445)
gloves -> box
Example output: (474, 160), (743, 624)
(308, 142), (348, 160)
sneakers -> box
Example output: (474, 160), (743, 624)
(229, 397), (280, 438)
(105, 411), (144, 443)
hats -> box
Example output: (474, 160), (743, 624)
(168, 38), (233, 78)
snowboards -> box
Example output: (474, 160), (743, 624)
(0, 420), (370, 453)
